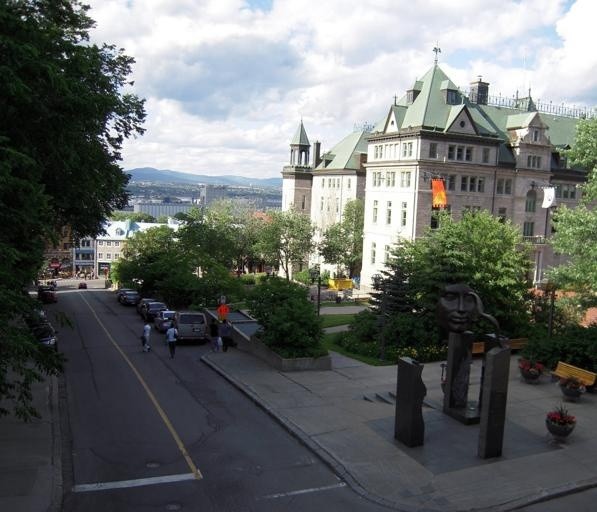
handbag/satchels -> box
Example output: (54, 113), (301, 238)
(174, 328), (178, 338)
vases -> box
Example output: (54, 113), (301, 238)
(561, 386), (582, 400)
(545, 419), (575, 438)
(521, 376), (540, 384)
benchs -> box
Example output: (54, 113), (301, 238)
(549, 361), (596, 385)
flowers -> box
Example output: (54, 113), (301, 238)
(516, 357), (544, 375)
(545, 402), (576, 424)
(558, 376), (587, 392)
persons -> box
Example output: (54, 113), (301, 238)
(210, 319), (219, 353)
(220, 319), (230, 353)
(154, 311), (179, 360)
(141, 321), (151, 352)
(439, 285), (478, 333)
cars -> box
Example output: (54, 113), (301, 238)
(38, 280), (57, 304)
(143, 302), (169, 321)
(28, 323), (58, 354)
(77, 282), (87, 289)
(136, 298), (154, 312)
(27, 307), (48, 326)
(153, 310), (176, 332)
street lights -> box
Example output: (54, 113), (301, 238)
(370, 272), (389, 361)
(540, 278), (556, 340)
(309, 269), (321, 319)
(264, 265), (272, 277)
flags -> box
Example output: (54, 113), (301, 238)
(431, 179), (446, 208)
(542, 187), (557, 209)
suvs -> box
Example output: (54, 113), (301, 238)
(174, 311), (208, 344)
(116, 288), (141, 305)
